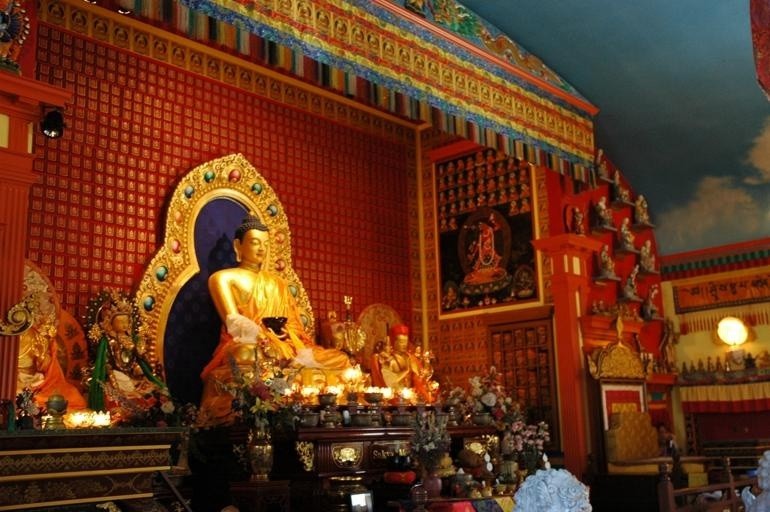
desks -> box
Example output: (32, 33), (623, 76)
(0, 424), (189, 510)
(293, 426), (499, 491)
(416, 494), (516, 512)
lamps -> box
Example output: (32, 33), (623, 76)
(41, 110), (66, 139)
